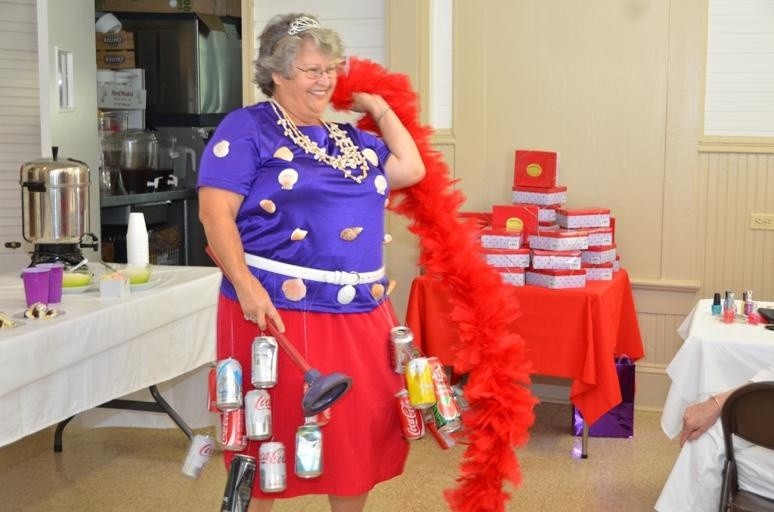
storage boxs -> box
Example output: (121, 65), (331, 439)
(95, 31), (148, 109)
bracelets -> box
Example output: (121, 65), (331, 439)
(372, 107), (391, 127)
(709, 393), (722, 411)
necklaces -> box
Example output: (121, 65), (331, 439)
(265, 97), (370, 186)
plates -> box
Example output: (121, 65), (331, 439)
(61, 285), (95, 295)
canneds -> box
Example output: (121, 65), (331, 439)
(220, 452), (257, 512)
(389, 325), (462, 450)
(206, 367), (223, 414)
(180, 431), (218, 480)
(245, 390), (271, 441)
(218, 408), (248, 451)
(215, 359), (244, 412)
(259, 442), (287, 492)
(293, 427), (323, 478)
(251, 334), (278, 390)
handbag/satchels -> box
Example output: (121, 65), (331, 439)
(572, 354), (635, 439)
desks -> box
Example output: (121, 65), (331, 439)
(0, 264), (222, 450)
(695, 296), (772, 401)
(406, 268), (629, 459)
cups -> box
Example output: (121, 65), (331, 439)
(21, 266), (52, 307)
(125, 212), (150, 264)
(36, 263), (64, 303)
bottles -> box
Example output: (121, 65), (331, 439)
(711, 290), (759, 324)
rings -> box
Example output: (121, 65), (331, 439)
(243, 314), (249, 321)
(249, 315), (256, 321)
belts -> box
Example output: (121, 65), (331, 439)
(243, 252), (386, 286)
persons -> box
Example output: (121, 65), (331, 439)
(194, 10), (429, 510)
(678, 361), (773, 511)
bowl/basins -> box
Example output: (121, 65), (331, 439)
(62, 270), (93, 287)
(112, 264), (152, 284)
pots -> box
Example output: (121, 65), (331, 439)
(106, 128), (159, 171)
(20, 145), (91, 245)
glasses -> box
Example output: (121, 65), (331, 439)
(295, 63), (342, 80)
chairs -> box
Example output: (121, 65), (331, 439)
(716, 379), (774, 512)
